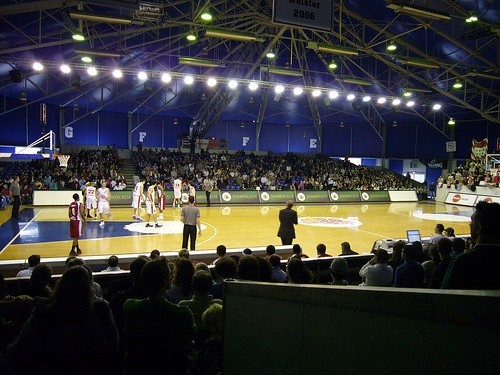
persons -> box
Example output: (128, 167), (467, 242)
(181, 197), (201, 251)
(429, 181), (435, 201)
(0, 144), (127, 211)
(145, 181), (163, 228)
(188, 181), (197, 207)
(68, 192), (83, 256)
(440, 201), (500, 290)
(0, 223), (500, 375)
(136, 141), (427, 201)
(204, 176), (214, 207)
(157, 182), (166, 219)
(173, 177), (182, 209)
(9, 175), (22, 218)
(276, 200), (298, 245)
(437, 158), (500, 191)
(95, 179), (111, 226)
(131, 178), (146, 222)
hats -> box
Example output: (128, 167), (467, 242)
(330, 258), (349, 273)
(444, 228), (454, 234)
(374, 249), (389, 260)
(437, 238), (452, 250)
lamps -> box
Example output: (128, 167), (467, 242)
(9, 4), (500, 124)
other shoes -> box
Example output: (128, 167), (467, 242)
(70, 251), (78, 256)
(133, 216), (143, 221)
(87, 215), (92, 218)
(99, 222), (105, 226)
(155, 223), (162, 227)
(146, 223), (153, 227)
(77, 249), (82, 254)
